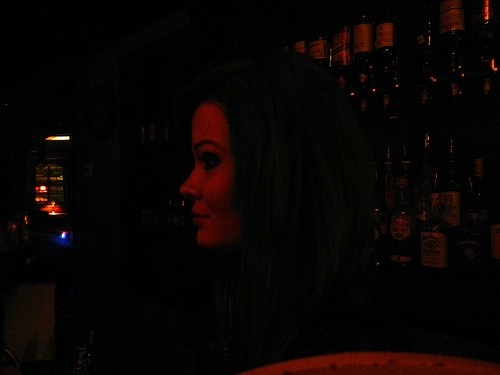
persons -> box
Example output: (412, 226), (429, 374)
(150, 50), (414, 375)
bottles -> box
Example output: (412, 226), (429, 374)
(291, 0), (500, 273)
